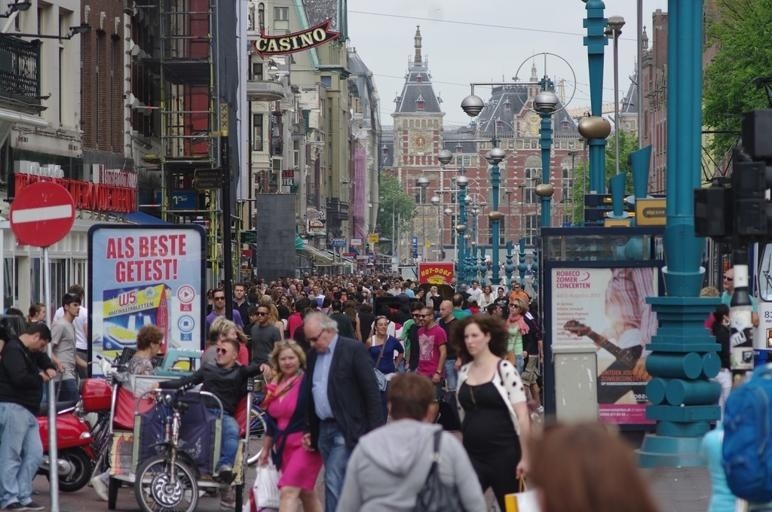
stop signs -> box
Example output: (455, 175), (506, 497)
(11, 180), (76, 249)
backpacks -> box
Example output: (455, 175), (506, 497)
(722, 366), (771, 502)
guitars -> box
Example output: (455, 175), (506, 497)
(564, 321), (643, 369)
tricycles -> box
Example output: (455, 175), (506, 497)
(109, 373), (252, 511)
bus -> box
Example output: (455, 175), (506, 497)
(697, 166), (772, 386)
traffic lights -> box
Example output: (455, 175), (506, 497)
(732, 161), (769, 243)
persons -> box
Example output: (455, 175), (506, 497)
(699, 268), (760, 431)
(89, 327), (163, 503)
(531, 420), (662, 512)
(296, 313), (385, 510)
(314, 269), (543, 425)
(328, 373), (487, 512)
(149, 274), (325, 511)
(0, 286), (87, 510)
(452, 316), (531, 511)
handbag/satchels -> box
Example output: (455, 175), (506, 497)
(252, 462), (280, 511)
(416, 462), (464, 512)
(505, 474), (545, 512)
(502, 351), (515, 366)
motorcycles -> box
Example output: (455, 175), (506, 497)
(33, 375), (114, 491)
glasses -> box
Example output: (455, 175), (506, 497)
(311, 329), (324, 341)
(256, 312), (267, 316)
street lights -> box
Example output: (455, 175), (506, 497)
(460, 53), (576, 229)
(416, 164), (480, 277)
(436, 120), (503, 285)
(605, 16), (626, 175)
(505, 137), (590, 240)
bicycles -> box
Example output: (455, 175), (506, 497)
(90, 356), (270, 468)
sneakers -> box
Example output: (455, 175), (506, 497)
(7, 503), (25, 511)
(220, 500), (244, 511)
(90, 477), (109, 501)
(212, 465), (232, 483)
(26, 502), (44, 511)
